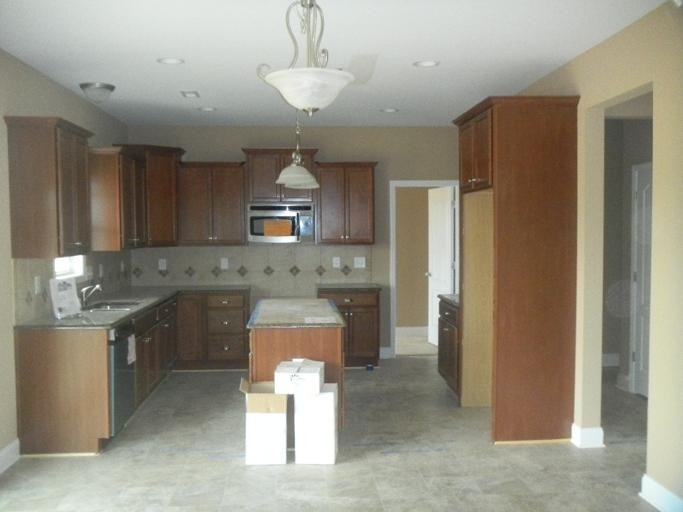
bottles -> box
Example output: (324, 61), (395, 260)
(366, 360), (373, 373)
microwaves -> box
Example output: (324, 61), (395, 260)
(249, 204), (315, 243)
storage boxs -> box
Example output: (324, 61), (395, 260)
(238, 358), (340, 467)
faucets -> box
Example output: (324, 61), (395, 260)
(80, 284), (103, 307)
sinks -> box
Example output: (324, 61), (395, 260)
(80, 300), (143, 313)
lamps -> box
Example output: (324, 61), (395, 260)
(78, 83), (114, 104)
(255, 0), (355, 190)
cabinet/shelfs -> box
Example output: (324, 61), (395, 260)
(177, 162), (246, 247)
(316, 161), (376, 244)
(176, 286), (205, 370)
(246, 296), (346, 383)
(243, 147), (318, 204)
(6, 115), (94, 260)
(15, 290), (175, 454)
(438, 294), (459, 395)
(453, 94), (578, 443)
(87, 144), (186, 252)
(315, 281), (379, 367)
(206, 284), (252, 369)
